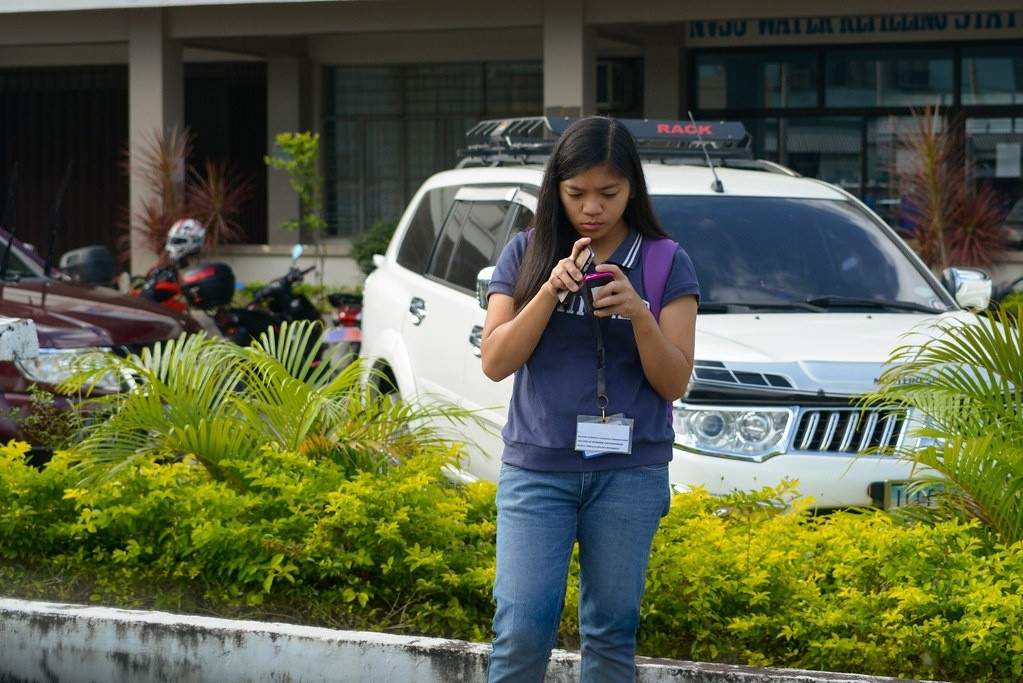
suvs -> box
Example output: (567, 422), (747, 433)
(357, 112), (1022, 532)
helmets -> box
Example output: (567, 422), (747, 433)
(164, 219), (207, 262)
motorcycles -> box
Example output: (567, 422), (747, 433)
(56, 243), (386, 395)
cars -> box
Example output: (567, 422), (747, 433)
(0, 296), (169, 448)
(0, 226), (207, 403)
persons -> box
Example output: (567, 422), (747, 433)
(480, 116), (700, 683)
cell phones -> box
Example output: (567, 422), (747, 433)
(585, 272), (617, 317)
(557, 245), (594, 304)
